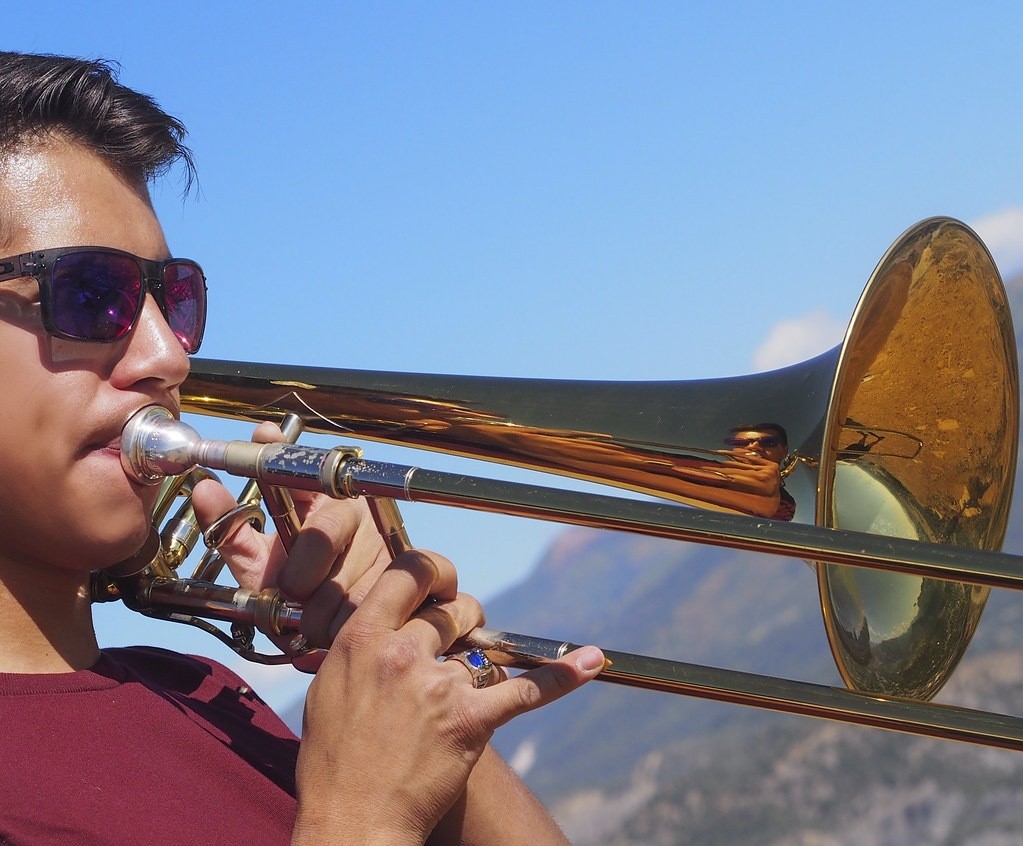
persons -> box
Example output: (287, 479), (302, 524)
(0, 50), (608, 846)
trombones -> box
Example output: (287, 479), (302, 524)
(83, 218), (1023, 752)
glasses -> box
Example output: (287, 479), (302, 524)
(0, 246), (207, 357)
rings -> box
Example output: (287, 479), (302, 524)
(444, 648), (494, 689)
